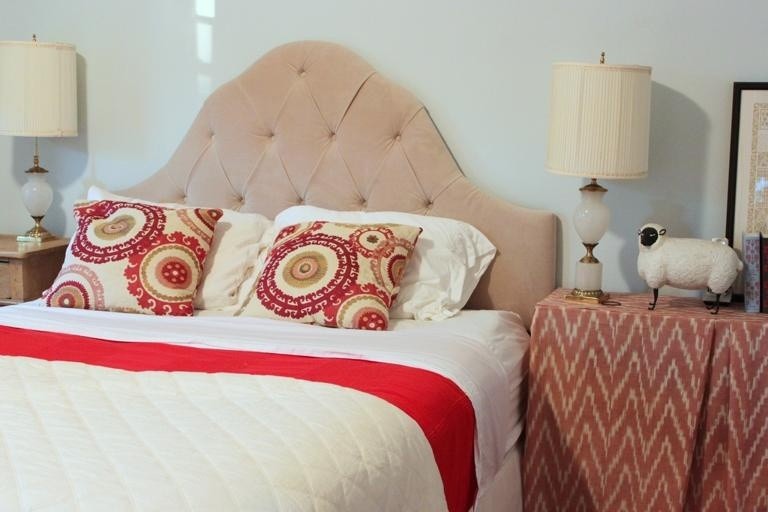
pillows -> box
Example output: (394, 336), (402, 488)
(238, 205), (497, 322)
(236, 219), (422, 331)
(38, 199), (223, 318)
(87, 184), (272, 316)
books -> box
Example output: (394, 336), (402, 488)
(740, 232), (762, 312)
(760, 235), (768, 313)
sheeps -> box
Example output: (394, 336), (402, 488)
(637, 225), (744, 315)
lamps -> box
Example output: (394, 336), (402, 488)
(544, 53), (652, 302)
(0, 32), (78, 243)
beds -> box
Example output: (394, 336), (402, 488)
(0, 42), (556, 512)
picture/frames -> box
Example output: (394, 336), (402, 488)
(724, 81), (768, 303)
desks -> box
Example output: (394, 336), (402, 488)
(523, 285), (767, 512)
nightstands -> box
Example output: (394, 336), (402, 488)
(0, 232), (71, 307)
